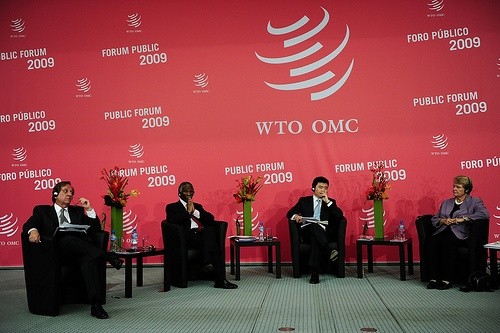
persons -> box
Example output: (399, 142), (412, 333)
(22, 181), (124, 319)
(165, 182), (238, 289)
(427, 176), (490, 290)
(286, 175), (343, 284)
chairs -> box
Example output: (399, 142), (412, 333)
(288, 210), (347, 278)
(416, 214), (490, 283)
(161, 219), (228, 292)
(22, 205), (110, 317)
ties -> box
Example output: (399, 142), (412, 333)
(313, 200), (320, 221)
(191, 213), (203, 230)
(59, 208), (69, 224)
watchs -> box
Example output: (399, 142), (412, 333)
(463, 216), (467, 222)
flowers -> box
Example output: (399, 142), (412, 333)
(101, 166), (141, 208)
(232, 174), (265, 204)
(365, 161), (392, 200)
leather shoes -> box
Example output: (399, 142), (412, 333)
(90, 304), (108, 319)
(107, 252), (124, 270)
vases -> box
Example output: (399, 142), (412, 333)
(111, 205), (123, 251)
(243, 199), (252, 236)
(374, 199), (383, 239)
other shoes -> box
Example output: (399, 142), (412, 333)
(438, 282), (453, 290)
(310, 272), (319, 284)
(201, 259), (215, 273)
(329, 248), (339, 262)
(427, 281), (439, 289)
(214, 278), (238, 289)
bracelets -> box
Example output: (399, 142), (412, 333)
(327, 200), (330, 204)
(87, 208), (92, 211)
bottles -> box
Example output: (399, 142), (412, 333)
(110, 231), (116, 252)
(131, 229), (138, 250)
(258, 221), (264, 242)
(399, 220), (405, 241)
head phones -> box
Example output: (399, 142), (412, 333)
(53, 184), (60, 198)
(466, 176), (472, 193)
(177, 182), (182, 196)
(312, 183), (315, 192)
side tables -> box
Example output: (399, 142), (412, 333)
(230, 236), (282, 280)
(107, 248), (171, 298)
(484, 241), (500, 280)
(356, 236), (413, 281)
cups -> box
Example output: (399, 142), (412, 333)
(116, 237), (123, 252)
(266, 227), (272, 241)
(393, 226), (398, 239)
(143, 235), (149, 251)
